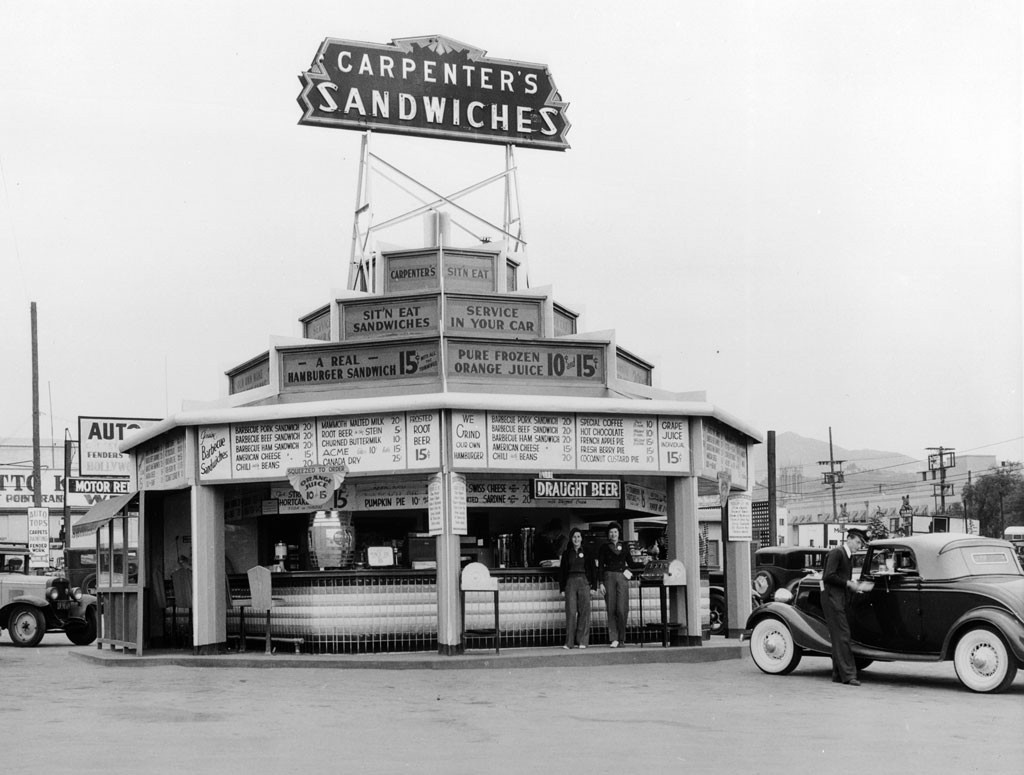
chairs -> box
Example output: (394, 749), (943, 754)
(151, 566), (288, 654)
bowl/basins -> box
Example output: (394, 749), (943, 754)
(854, 581), (873, 591)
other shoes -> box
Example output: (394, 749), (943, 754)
(578, 645), (587, 649)
(563, 643), (573, 649)
(609, 641), (619, 647)
(619, 641), (624, 647)
(832, 680), (842, 682)
(845, 679), (861, 686)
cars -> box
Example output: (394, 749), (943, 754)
(708, 545), (888, 635)
(43, 546), (138, 613)
(0, 540), (98, 648)
(739, 532), (1024, 694)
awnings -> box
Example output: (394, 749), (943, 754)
(72, 490), (140, 538)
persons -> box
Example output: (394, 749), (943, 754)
(597, 521), (637, 649)
(820, 528), (870, 687)
(558, 527), (596, 650)
(657, 533), (668, 560)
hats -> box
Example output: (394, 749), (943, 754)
(847, 528), (870, 544)
